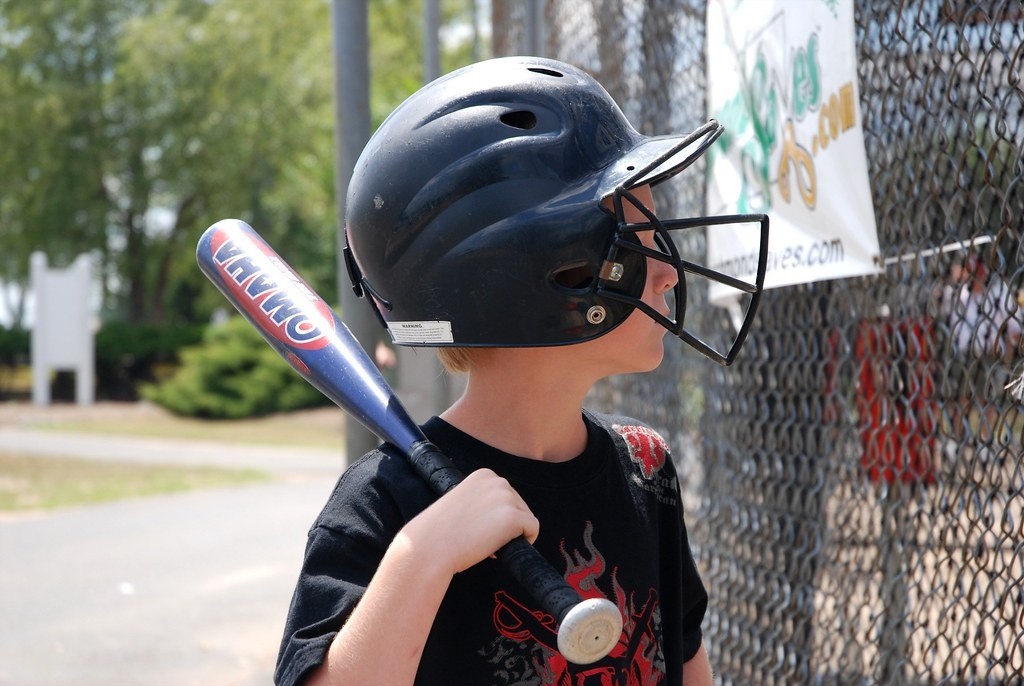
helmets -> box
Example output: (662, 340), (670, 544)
(343, 55), (725, 349)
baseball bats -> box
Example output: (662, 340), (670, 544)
(193, 214), (625, 667)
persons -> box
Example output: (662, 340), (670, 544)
(946, 239), (1024, 447)
(272, 56), (744, 686)
